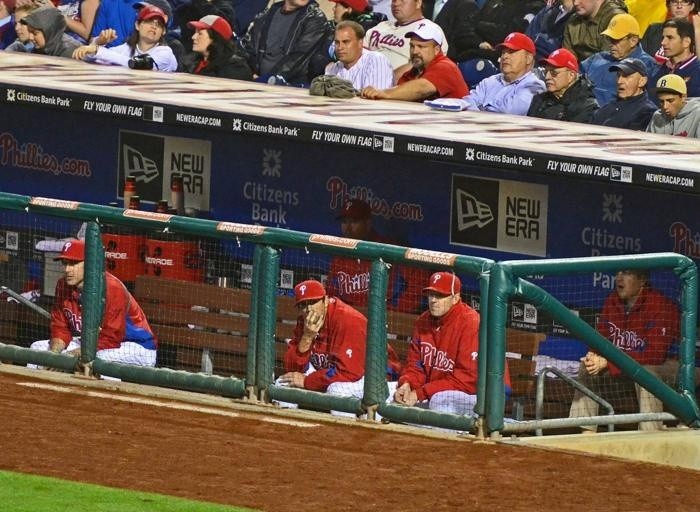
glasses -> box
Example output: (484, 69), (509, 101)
(541, 68), (572, 77)
(669, 1), (692, 7)
(538, 48), (580, 74)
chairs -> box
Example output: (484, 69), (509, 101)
(456, 60), (498, 91)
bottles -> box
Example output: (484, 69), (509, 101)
(106, 172), (186, 216)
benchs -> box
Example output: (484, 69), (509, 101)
(135, 277), (545, 420)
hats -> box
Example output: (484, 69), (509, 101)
(494, 30), (538, 57)
(607, 57), (649, 78)
(52, 238), (87, 263)
(420, 270), (463, 297)
(186, 14), (233, 42)
(332, 197), (374, 222)
(291, 278), (327, 308)
(654, 73), (688, 96)
(599, 13), (641, 41)
(327, 0), (370, 15)
(403, 21), (445, 48)
(131, 0), (173, 25)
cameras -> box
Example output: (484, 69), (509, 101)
(129, 54), (153, 69)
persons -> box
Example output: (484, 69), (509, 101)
(273, 279), (401, 417)
(326, 199), (428, 315)
(569, 268), (700, 433)
(390, 271), (512, 435)
(326, 2), (700, 138)
(25, 239), (159, 383)
(0, 0), (328, 87)
